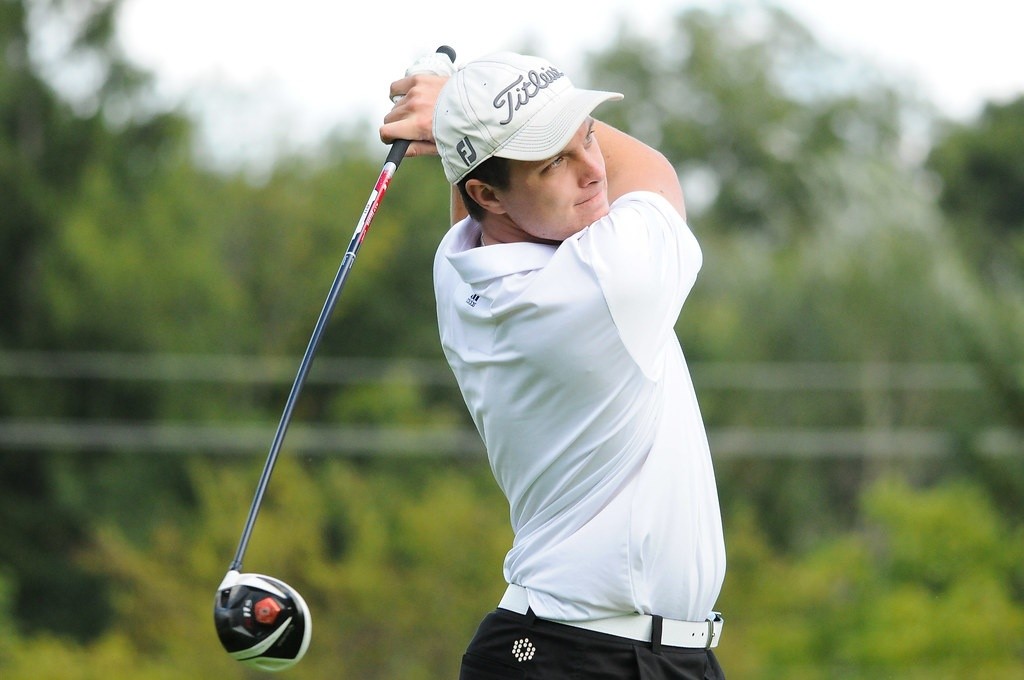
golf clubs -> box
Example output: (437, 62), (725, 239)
(213, 45), (457, 672)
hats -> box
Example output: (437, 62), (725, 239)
(432, 52), (623, 184)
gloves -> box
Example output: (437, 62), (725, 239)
(392, 51), (459, 104)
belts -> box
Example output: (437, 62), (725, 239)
(498, 585), (725, 650)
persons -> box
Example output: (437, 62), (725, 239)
(380, 51), (727, 680)
